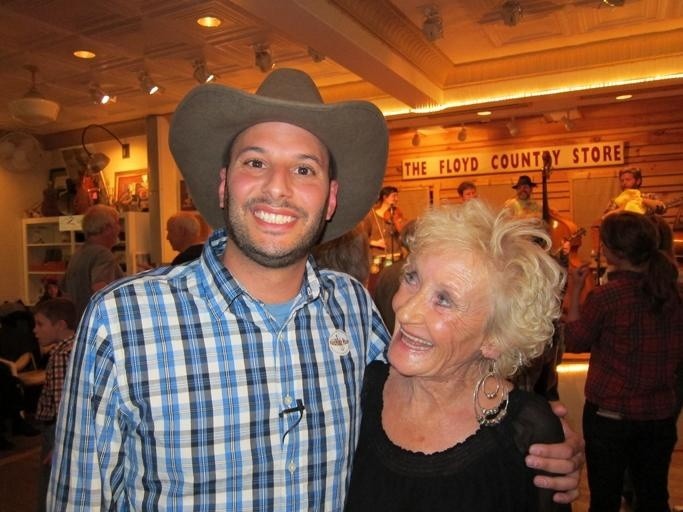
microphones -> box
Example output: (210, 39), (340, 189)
(390, 204), (394, 214)
(282, 398), (304, 443)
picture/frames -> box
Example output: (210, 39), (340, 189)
(132, 251), (152, 274)
(49, 167), (67, 192)
(114, 170), (148, 201)
(177, 178), (199, 215)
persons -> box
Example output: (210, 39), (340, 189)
(0, 203), (212, 455)
(310, 162), (680, 340)
(343, 196), (571, 509)
(31, 295), (77, 511)
(45, 122), (587, 511)
(567, 207), (683, 511)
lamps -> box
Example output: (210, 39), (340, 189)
(254, 46), (273, 73)
(424, 6), (444, 38)
(138, 70), (159, 94)
(81, 124), (129, 175)
(192, 60), (216, 84)
(412, 109), (575, 145)
(500, 1), (522, 26)
(89, 83), (110, 104)
(8, 66), (60, 126)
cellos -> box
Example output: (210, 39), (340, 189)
(533, 151), (596, 322)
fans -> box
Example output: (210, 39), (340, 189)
(0, 131), (42, 171)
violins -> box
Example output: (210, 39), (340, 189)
(384, 206), (409, 233)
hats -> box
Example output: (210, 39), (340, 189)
(168, 67), (388, 246)
(512, 176), (536, 189)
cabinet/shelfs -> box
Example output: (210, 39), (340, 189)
(21, 210), (151, 307)
